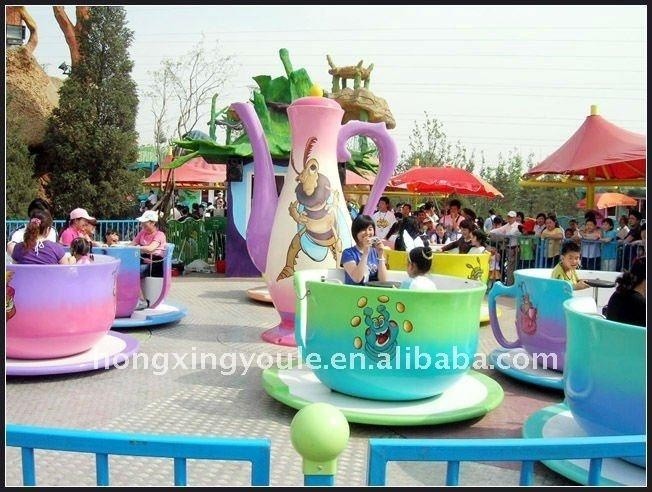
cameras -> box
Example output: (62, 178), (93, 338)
(371, 236), (379, 244)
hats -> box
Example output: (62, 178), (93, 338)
(70, 208), (95, 221)
(508, 211), (516, 217)
(136, 211), (158, 222)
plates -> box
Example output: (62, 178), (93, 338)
(524, 401), (646, 486)
(111, 303), (188, 328)
(242, 284), (275, 306)
(6, 331), (140, 375)
(488, 346), (564, 391)
(478, 303), (501, 326)
(261, 359), (505, 425)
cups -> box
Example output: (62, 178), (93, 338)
(7, 251), (121, 363)
(488, 268), (626, 374)
(293, 267), (488, 401)
(561, 296), (647, 471)
(62, 242), (175, 317)
(383, 247), (492, 285)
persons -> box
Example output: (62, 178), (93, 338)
(372, 196), (646, 290)
(58, 208), (96, 246)
(79, 217), (101, 247)
(7, 198), (59, 258)
(128, 210), (169, 310)
(102, 230), (121, 247)
(68, 236), (95, 265)
(605, 257), (646, 327)
(142, 191), (228, 223)
(11, 210), (68, 265)
(340, 215), (388, 285)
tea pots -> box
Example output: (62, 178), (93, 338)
(231, 86), (396, 346)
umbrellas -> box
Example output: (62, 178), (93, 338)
(143, 155), (228, 190)
(577, 193), (637, 218)
(386, 167), (504, 228)
(519, 105), (646, 212)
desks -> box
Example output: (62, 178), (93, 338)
(577, 279), (617, 305)
(365, 280), (401, 287)
